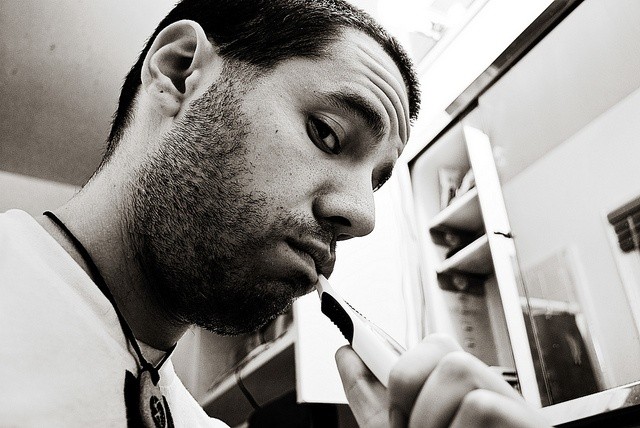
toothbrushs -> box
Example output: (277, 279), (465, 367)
(311, 274), (405, 394)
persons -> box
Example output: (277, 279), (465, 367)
(0, 0), (556, 428)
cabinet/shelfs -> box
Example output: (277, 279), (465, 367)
(409, 121), (543, 428)
(470, 45), (640, 428)
(191, 308), (294, 426)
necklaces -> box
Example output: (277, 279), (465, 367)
(39, 207), (181, 428)
